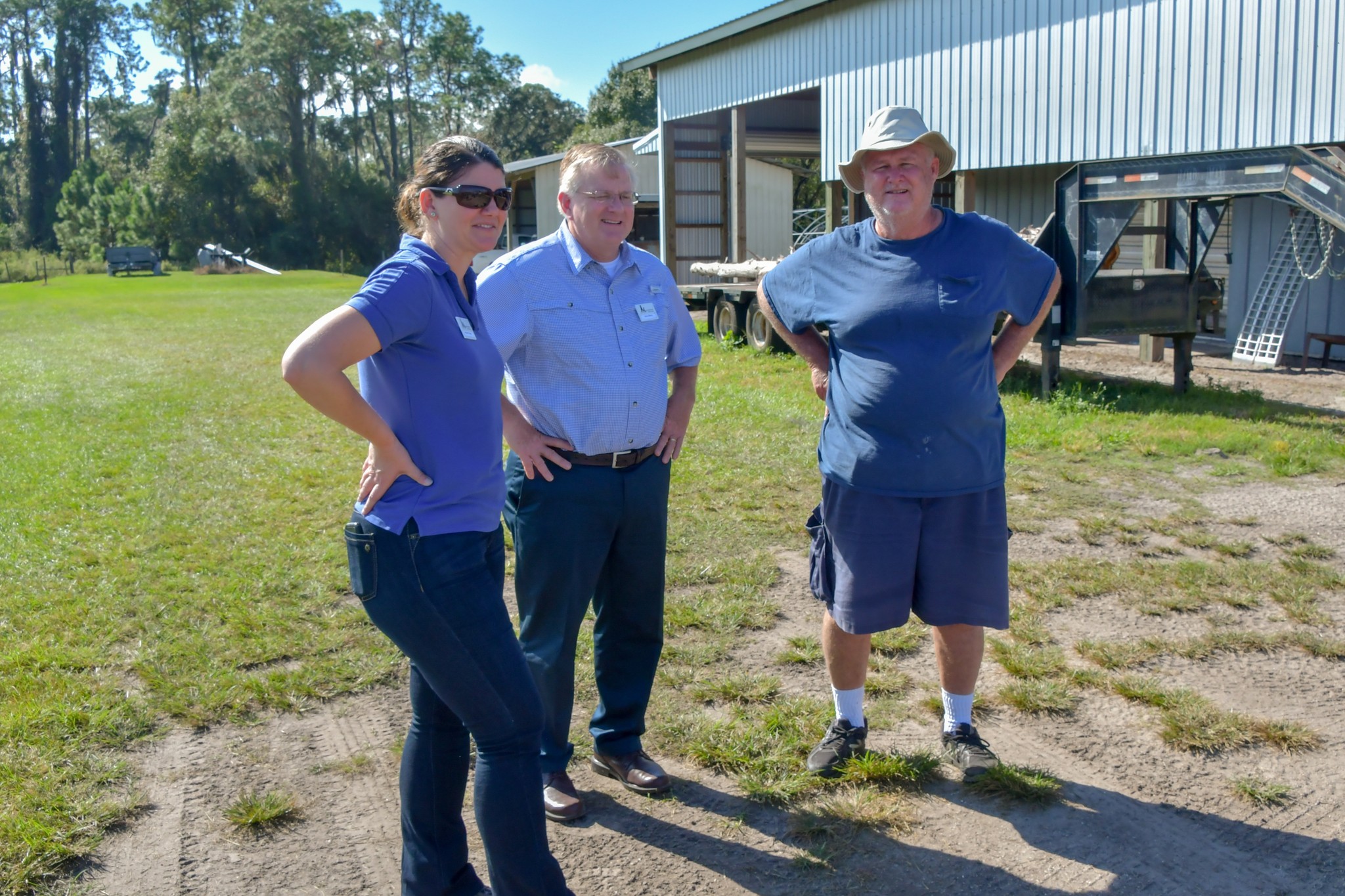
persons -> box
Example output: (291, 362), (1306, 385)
(474, 143), (702, 821)
(280, 133), (582, 896)
(756, 106), (1063, 774)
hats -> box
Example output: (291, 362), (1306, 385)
(838, 105), (957, 194)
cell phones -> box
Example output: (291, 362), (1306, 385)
(345, 521), (364, 534)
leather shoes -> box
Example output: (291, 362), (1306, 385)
(590, 746), (672, 795)
(542, 767), (586, 822)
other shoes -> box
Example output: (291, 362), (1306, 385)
(474, 885), (493, 896)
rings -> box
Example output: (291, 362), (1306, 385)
(670, 438), (677, 442)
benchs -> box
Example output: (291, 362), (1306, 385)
(103, 246), (159, 277)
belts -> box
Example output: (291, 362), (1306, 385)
(547, 443), (658, 469)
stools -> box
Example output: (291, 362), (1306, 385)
(1301, 332), (1345, 374)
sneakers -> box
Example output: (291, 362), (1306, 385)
(941, 722), (1001, 782)
(807, 715), (868, 778)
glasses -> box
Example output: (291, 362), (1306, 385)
(576, 189), (641, 206)
(413, 184), (513, 211)
